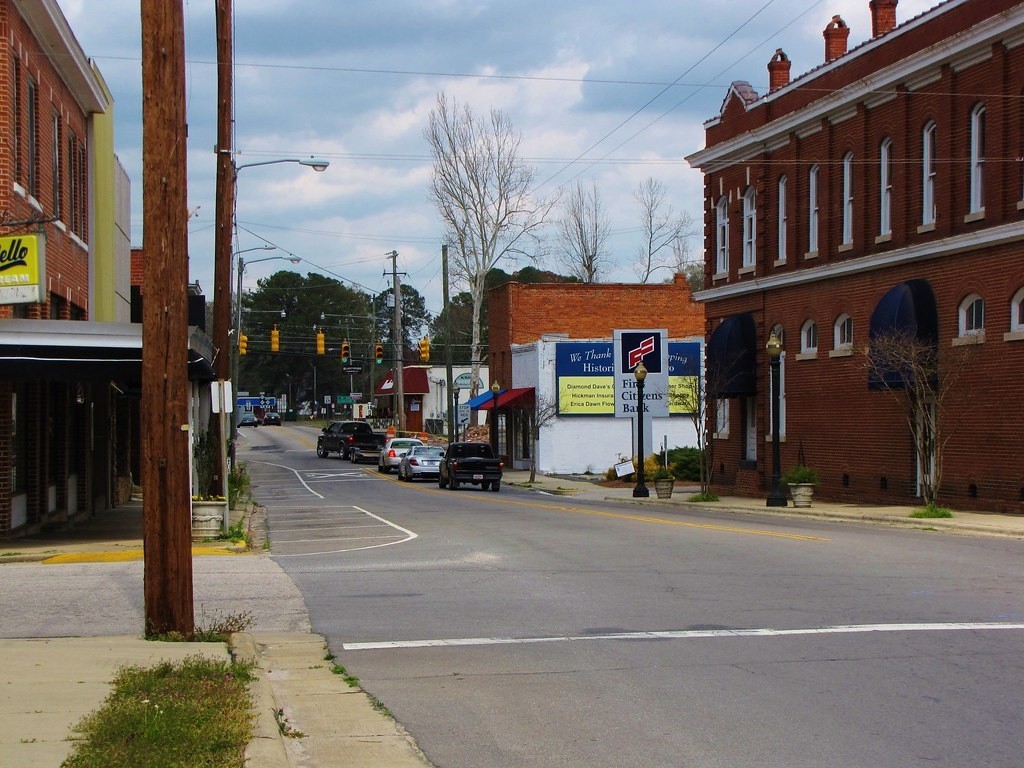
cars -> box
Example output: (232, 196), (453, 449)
(237, 412), (258, 428)
(262, 412), (281, 426)
(397, 445), (446, 482)
(378, 438), (424, 474)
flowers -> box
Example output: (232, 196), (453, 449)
(190, 495), (227, 502)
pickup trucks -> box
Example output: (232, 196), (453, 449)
(439, 441), (504, 492)
(316, 421), (386, 460)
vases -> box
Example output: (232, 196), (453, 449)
(191, 502), (226, 540)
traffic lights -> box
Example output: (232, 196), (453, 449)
(342, 344), (349, 363)
(417, 333), (429, 362)
(239, 332), (247, 356)
(376, 345), (383, 365)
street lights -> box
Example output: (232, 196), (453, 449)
(209, 154), (330, 499)
(228, 244), (276, 301)
(766, 328), (790, 508)
(491, 378), (502, 457)
(230, 254), (302, 442)
(320, 292), (388, 405)
(451, 380), (460, 443)
(633, 359), (650, 497)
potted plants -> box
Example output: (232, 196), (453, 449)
(653, 470), (675, 500)
(786, 466), (818, 509)
